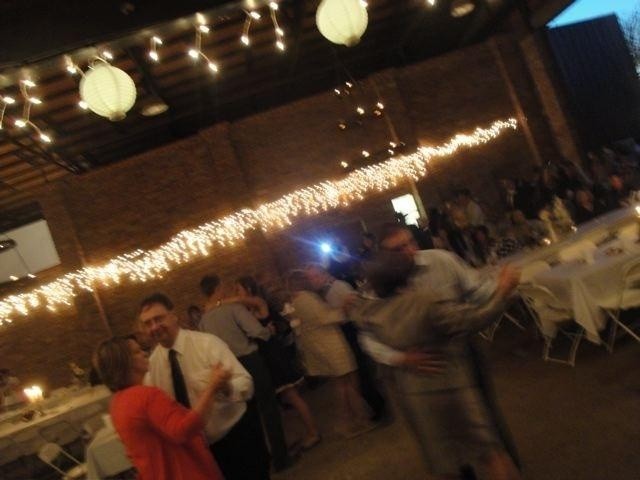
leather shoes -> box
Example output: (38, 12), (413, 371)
(272, 412), (396, 474)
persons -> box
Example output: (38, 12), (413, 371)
(196, 275), (301, 474)
(205, 276), (322, 452)
(137, 292), (271, 480)
(95, 333), (232, 480)
(286, 139), (640, 480)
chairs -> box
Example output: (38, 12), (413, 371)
(37, 410), (107, 480)
(473, 191), (640, 367)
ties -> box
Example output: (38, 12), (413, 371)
(169, 349), (191, 409)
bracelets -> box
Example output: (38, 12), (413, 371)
(216, 297), (221, 307)
